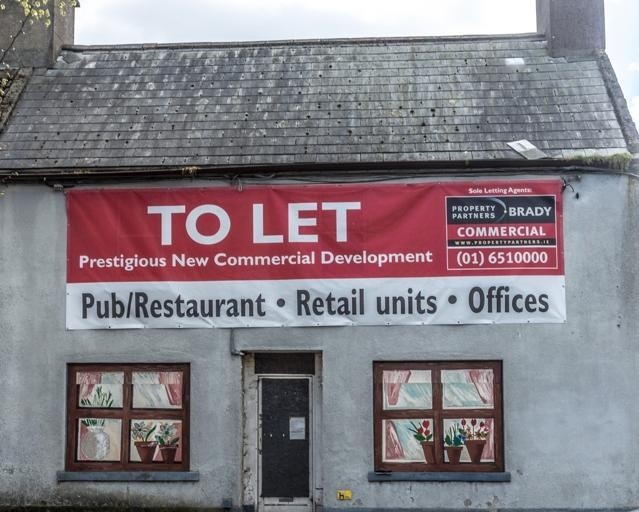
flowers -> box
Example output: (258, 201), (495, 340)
(132, 421), (181, 446)
(406, 419), (490, 446)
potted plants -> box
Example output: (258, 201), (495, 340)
(80, 385), (114, 461)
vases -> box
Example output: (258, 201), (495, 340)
(420, 440), (487, 464)
(134, 440), (178, 464)
